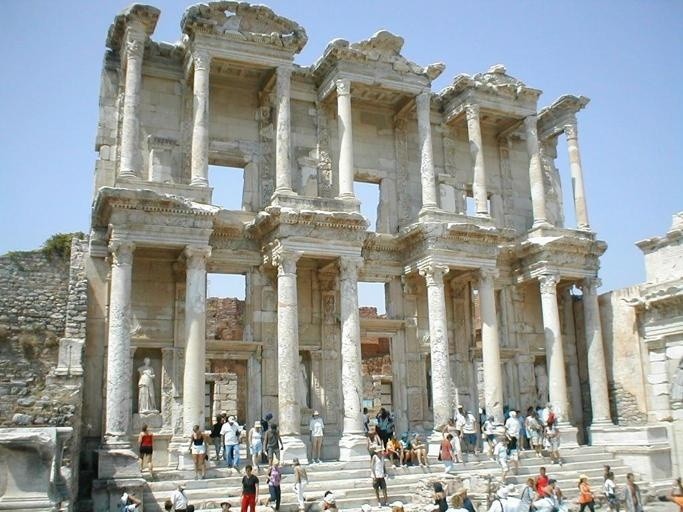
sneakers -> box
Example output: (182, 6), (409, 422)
(193, 473), (207, 480)
(311, 458), (324, 465)
(391, 462), (430, 469)
(216, 454), (242, 470)
(535, 452), (563, 466)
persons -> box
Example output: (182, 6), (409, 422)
(136, 356), (162, 414)
(299, 354), (309, 408)
(535, 360), (549, 402)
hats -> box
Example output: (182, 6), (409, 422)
(323, 492), (337, 505)
(178, 481), (188, 489)
(454, 487), (468, 496)
(292, 457), (300, 465)
(313, 411), (321, 417)
(216, 410), (277, 434)
(373, 445), (386, 453)
(496, 482), (522, 500)
(444, 400), (559, 422)
(433, 482), (443, 493)
(389, 501), (404, 509)
(577, 473), (589, 482)
(548, 479), (558, 485)
